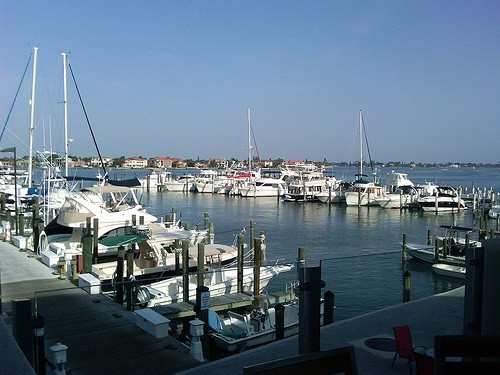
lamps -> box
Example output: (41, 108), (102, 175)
(64, 250), (74, 261)
(50, 342), (68, 365)
(189, 318), (206, 337)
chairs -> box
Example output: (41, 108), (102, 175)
(391, 326), (435, 375)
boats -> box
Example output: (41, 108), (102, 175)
(0, 47), (214, 261)
(399, 241), (466, 266)
(91, 244), (247, 286)
(131, 262), (296, 307)
(207, 296), (337, 353)
(436, 235), (483, 248)
(216, 108), (468, 211)
(432, 262), (466, 281)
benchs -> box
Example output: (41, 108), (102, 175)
(78, 273), (102, 295)
(41, 251), (59, 268)
(134, 308), (171, 338)
(14, 236), (26, 249)
(228, 311), (254, 336)
